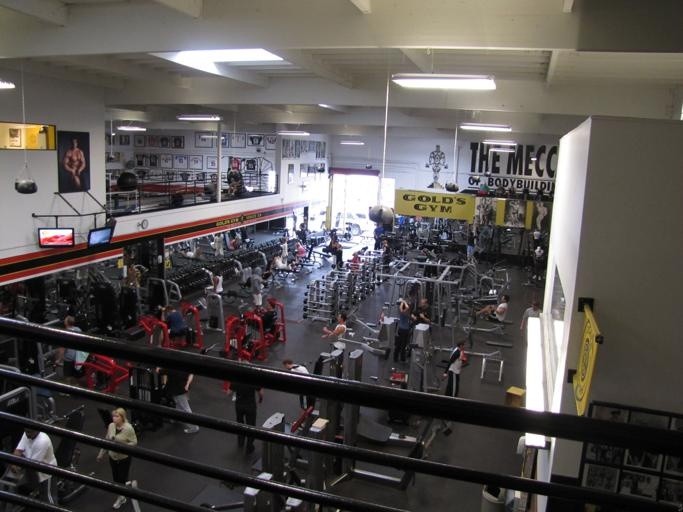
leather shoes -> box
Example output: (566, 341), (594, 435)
(247, 446), (255, 454)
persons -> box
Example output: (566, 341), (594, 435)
(442, 340), (468, 397)
(223, 379), (263, 457)
(319, 311), (347, 368)
(281, 358), (316, 414)
(393, 297), (413, 364)
(61, 136), (85, 190)
(153, 251), (281, 366)
(344, 218), (468, 298)
(475, 196), (548, 230)
(465, 232), (477, 264)
(519, 299), (542, 330)
(53, 316), (83, 398)
(530, 227), (545, 281)
(165, 225), (257, 263)
(473, 294), (508, 323)
(270, 222), (347, 283)
(408, 298), (433, 335)
(94, 408), (137, 510)
(8, 418), (59, 506)
(153, 365), (199, 434)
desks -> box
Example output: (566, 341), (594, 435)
(106, 187), (139, 215)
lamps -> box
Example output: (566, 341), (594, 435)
(176, 110), (224, 121)
(137, 219), (148, 230)
(116, 124), (147, 132)
(390, 69), (517, 153)
(14, 64), (38, 193)
(276, 129), (311, 136)
(339, 140), (365, 145)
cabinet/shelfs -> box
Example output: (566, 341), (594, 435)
(505, 386), (525, 408)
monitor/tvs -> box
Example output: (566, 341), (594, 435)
(88, 227), (112, 248)
(38, 228), (74, 248)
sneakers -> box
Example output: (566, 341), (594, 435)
(185, 427), (199, 434)
(112, 495), (126, 510)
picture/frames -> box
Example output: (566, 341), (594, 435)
(577, 400), (683, 505)
(106, 132), (282, 185)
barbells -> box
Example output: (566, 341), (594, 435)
(302, 249), (390, 325)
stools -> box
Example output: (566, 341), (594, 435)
(480, 355), (504, 384)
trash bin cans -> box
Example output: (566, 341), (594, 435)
(481, 484), (507, 512)
